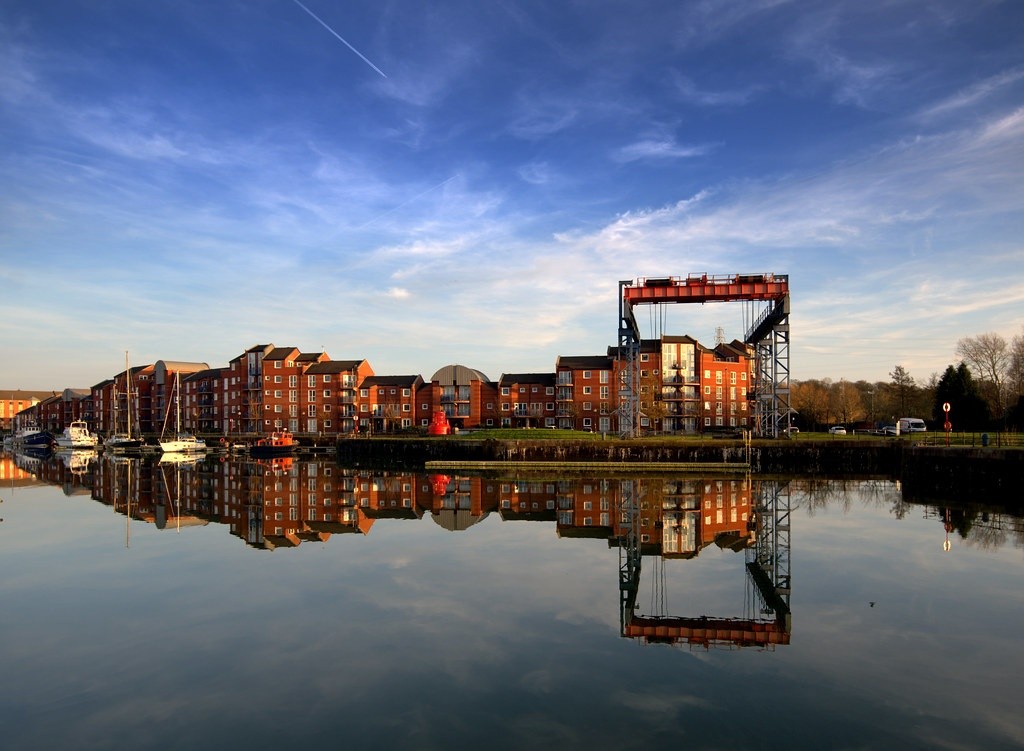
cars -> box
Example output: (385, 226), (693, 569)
(545, 425), (558, 429)
(563, 426), (575, 431)
(582, 427), (593, 433)
(783, 426), (800, 433)
(829, 426), (846, 436)
(882, 425), (897, 434)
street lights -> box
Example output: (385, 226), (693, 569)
(593, 408), (597, 434)
(301, 411), (306, 432)
(237, 411), (241, 435)
(672, 407), (678, 436)
(370, 410), (375, 436)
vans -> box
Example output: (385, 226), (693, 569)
(899, 417), (927, 433)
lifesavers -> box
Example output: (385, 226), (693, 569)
(943, 522), (953, 533)
(942, 402), (951, 412)
(219, 457), (225, 463)
(942, 540), (951, 552)
(219, 438), (225, 443)
(944, 421), (952, 431)
(93, 439), (96, 442)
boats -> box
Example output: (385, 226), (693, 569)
(249, 426), (300, 453)
(56, 447), (100, 485)
(55, 418), (99, 449)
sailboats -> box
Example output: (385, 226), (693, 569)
(107, 448), (144, 548)
(156, 370), (207, 453)
(103, 351), (144, 452)
(158, 451), (207, 535)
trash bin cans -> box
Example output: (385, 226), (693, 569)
(982, 434), (989, 447)
